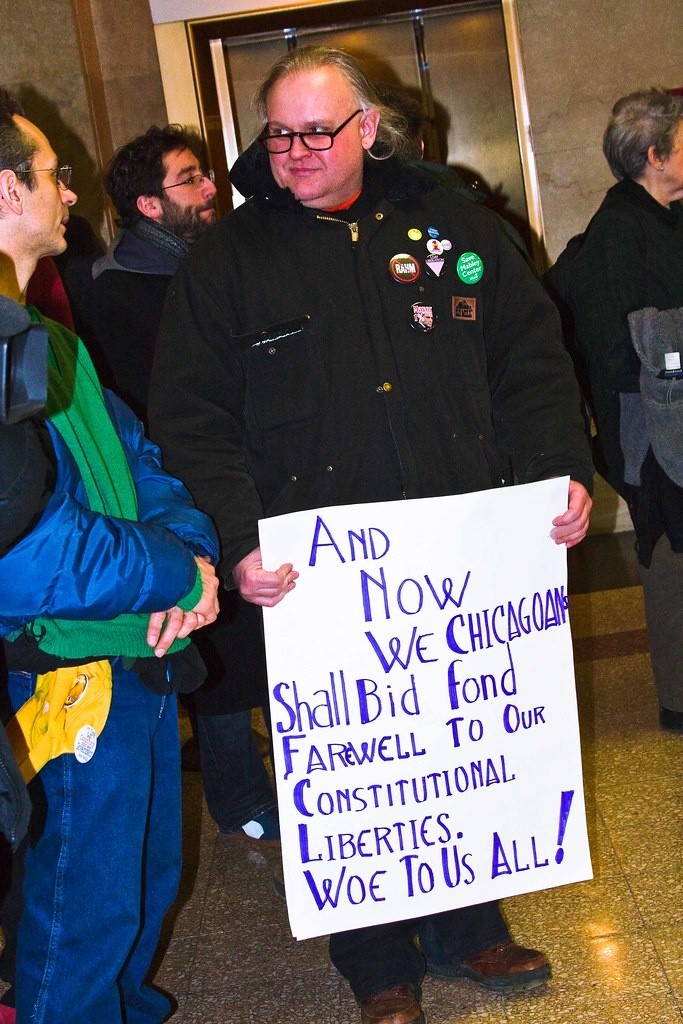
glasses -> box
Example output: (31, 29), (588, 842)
(2, 165), (71, 199)
(257, 108), (369, 154)
(145, 174), (207, 195)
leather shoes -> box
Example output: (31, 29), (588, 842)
(420, 941), (551, 992)
(361, 983), (424, 1024)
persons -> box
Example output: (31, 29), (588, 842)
(26, 226), (106, 378)
(85, 129), (279, 848)
(0, 96), (219, 1024)
(576, 88), (683, 732)
(147, 44), (595, 1024)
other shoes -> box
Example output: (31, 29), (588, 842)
(219, 805), (281, 848)
(658, 706), (683, 733)
